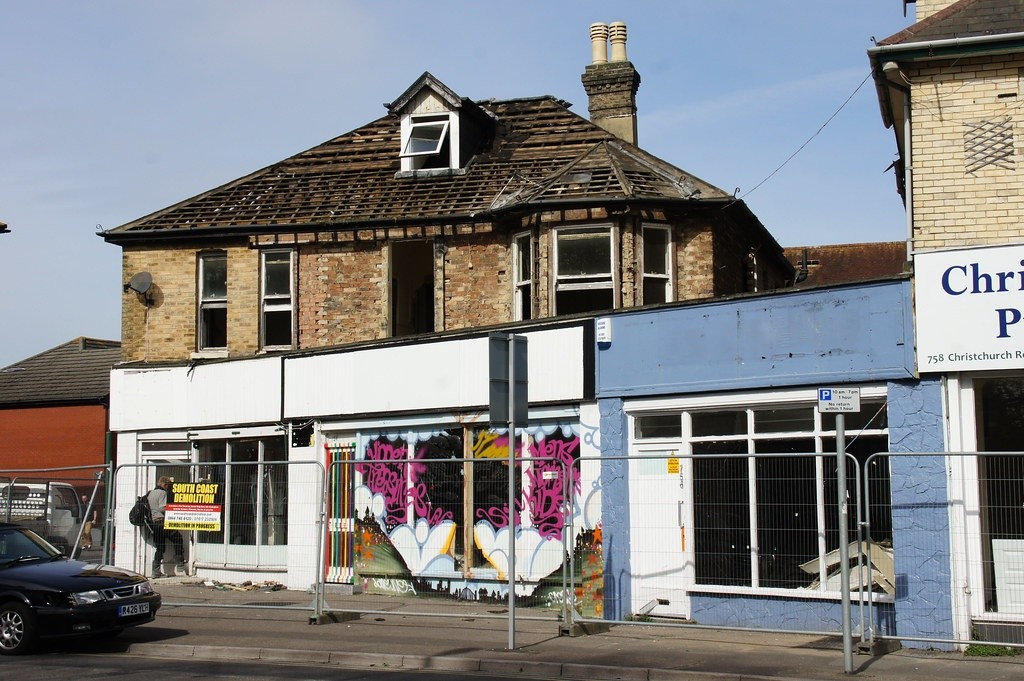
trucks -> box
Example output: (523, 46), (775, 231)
(0, 478), (94, 559)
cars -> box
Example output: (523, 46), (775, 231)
(0, 521), (162, 658)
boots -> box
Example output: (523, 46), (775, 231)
(176, 554), (186, 572)
(151, 561), (169, 579)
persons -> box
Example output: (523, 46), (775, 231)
(146, 475), (185, 579)
(80, 494), (98, 550)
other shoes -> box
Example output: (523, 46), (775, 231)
(88, 544), (91, 549)
(82, 546), (85, 550)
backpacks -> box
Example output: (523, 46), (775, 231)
(129, 490), (153, 525)
